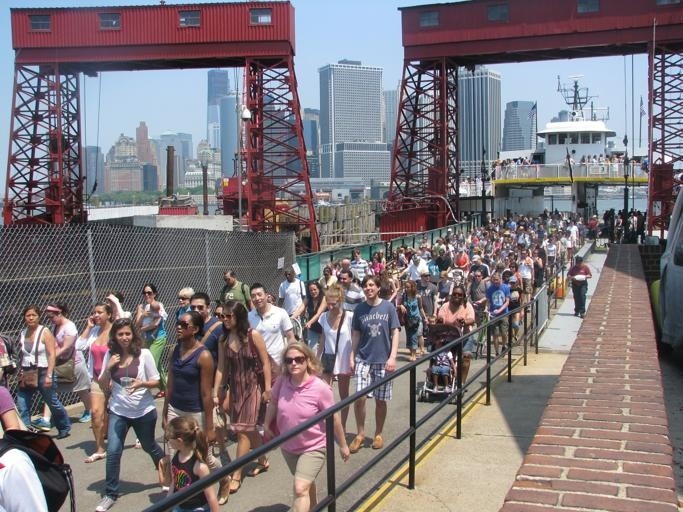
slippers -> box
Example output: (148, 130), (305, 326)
(156, 392), (165, 397)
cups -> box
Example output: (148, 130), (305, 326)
(120, 376), (133, 393)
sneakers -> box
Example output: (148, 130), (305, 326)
(30, 418), (51, 431)
(373, 434), (382, 449)
(349, 434), (365, 453)
(135, 439), (143, 449)
(217, 475), (231, 504)
(83, 451), (106, 462)
(79, 410), (91, 423)
(96, 496), (114, 511)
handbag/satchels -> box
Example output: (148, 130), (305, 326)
(53, 358), (76, 383)
(407, 318), (419, 329)
(256, 353), (278, 385)
(17, 366), (39, 390)
(158, 455), (171, 485)
(321, 353), (336, 374)
(397, 308), (406, 325)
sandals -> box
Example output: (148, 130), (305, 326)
(230, 478), (241, 493)
(246, 462), (268, 476)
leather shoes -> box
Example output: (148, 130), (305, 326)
(57, 430), (67, 439)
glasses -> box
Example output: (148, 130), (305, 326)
(452, 292), (463, 297)
(142, 291), (234, 329)
(285, 357), (306, 364)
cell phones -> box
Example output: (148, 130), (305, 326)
(215, 300), (222, 306)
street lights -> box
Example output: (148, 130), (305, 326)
(235, 101), (251, 229)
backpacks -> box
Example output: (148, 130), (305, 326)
(0, 430), (75, 512)
(0, 335), (18, 374)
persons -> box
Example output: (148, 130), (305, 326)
(95, 318), (165, 511)
(213, 300), (271, 494)
(349, 275), (402, 453)
(264, 343), (351, 512)
(74, 301), (143, 463)
(1, 268), (403, 439)
(312, 209), (601, 393)
(460, 156), (642, 196)
(0, 450), (50, 512)
(602, 208), (645, 244)
(166, 416), (220, 512)
(162, 311), (232, 506)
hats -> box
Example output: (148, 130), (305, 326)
(473, 247), (481, 252)
(419, 270), (429, 275)
(509, 276), (517, 282)
(472, 255), (480, 261)
(504, 231), (510, 234)
(502, 269), (513, 278)
(412, 254), (420, 261)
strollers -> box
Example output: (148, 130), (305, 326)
(419, 321), (466, 402)
